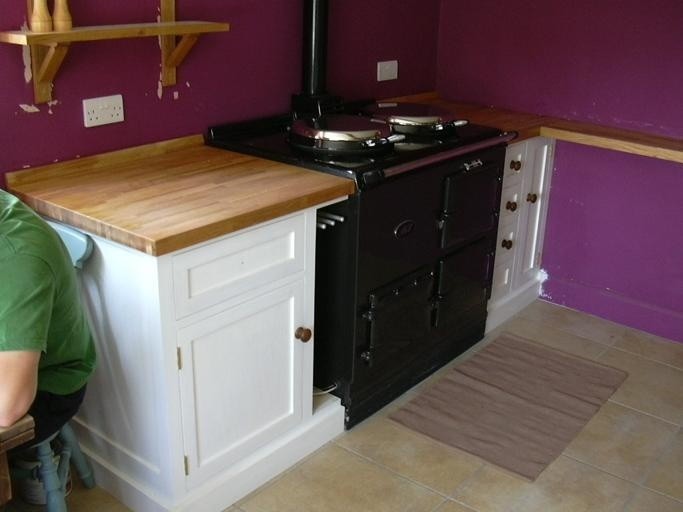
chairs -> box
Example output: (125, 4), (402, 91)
(28, 220), (96, 512)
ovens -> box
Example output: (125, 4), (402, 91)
(348, 140), (509, 408)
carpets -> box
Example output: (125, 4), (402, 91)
(384, 329), (631, 484)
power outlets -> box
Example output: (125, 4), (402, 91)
(82, 94), (125, 129)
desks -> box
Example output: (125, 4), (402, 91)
(0, 413), (35, 512)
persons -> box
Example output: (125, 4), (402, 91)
(0, 190), (98, 454)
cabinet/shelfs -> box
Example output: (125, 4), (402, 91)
(484, 136), (558, 336)
(39, 194), (348, 512)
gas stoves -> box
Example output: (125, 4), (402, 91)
(224, 102), (511, 187)
